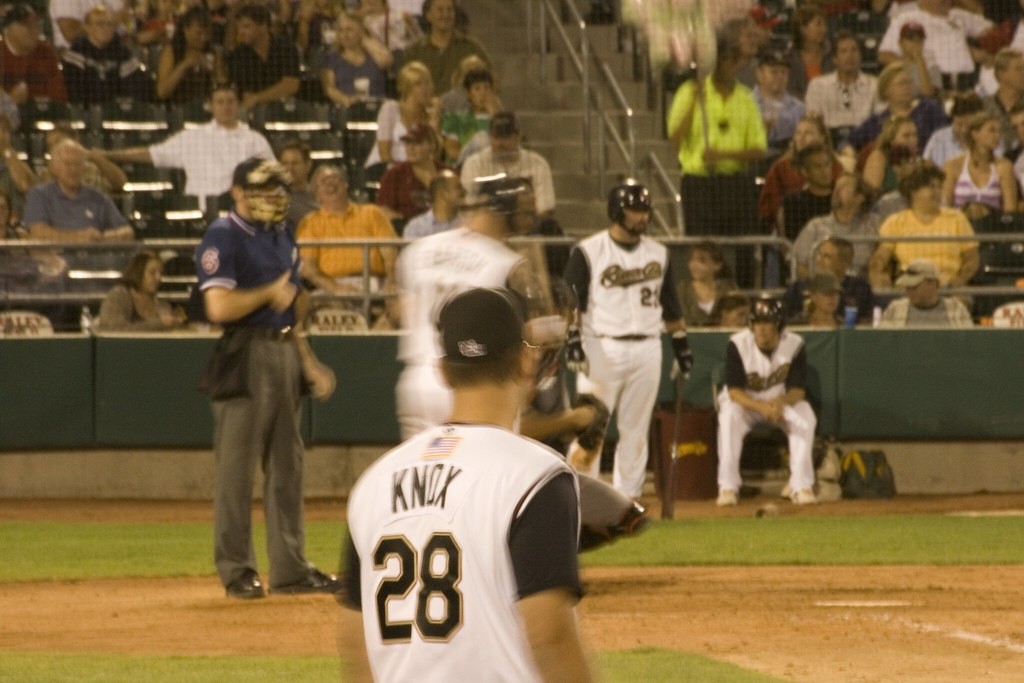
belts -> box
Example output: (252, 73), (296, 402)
(251, 324), (294, 343)
(615, 334), (648, 341)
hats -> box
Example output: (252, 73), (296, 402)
(231, 157), (289, 190)
(400, 123), (433, 142)
(901, 23), (925, 39)
(488, 111), (518, 137)
(438, 286), (528, 362)
(894, 260), (937, 288)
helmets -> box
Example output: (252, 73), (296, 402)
(607, 183), (651, 222)
(747, 294), (786, 331)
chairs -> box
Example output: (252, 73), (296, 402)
(712, 361), (827, 499)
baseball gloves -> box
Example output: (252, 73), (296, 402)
(572, 393), (611, 450)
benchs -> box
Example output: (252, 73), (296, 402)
(0, 40), (383, 291)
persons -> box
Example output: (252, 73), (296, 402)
(197, 158), (338, 597)
(336, 285), (609, 683)
(0, 1), (1024, 506)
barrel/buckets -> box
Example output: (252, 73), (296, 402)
(652, 410), (716, 499)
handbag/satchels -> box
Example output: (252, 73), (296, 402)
(841, 449), (895, 498)
(199, 327), (253, 403)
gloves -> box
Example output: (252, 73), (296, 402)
(563, 325), (588, 373)
(672, 334), (694, 380)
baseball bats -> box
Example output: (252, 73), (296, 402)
(661, 372), (687, 519)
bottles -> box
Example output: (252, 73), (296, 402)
(80, 308), (93, 335)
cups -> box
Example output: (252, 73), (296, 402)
(355, 79), (369, 102)
(846, 310), (857, 328)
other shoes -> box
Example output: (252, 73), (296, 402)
(273, 567), (338, 592)
(789, 491), (819, 506)
(224, 570), (267, 599)
(716, 492), (738, 507)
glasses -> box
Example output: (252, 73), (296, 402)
(842, 89), (851, 109)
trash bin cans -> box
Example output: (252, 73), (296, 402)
(651, 409), (720, 500)
